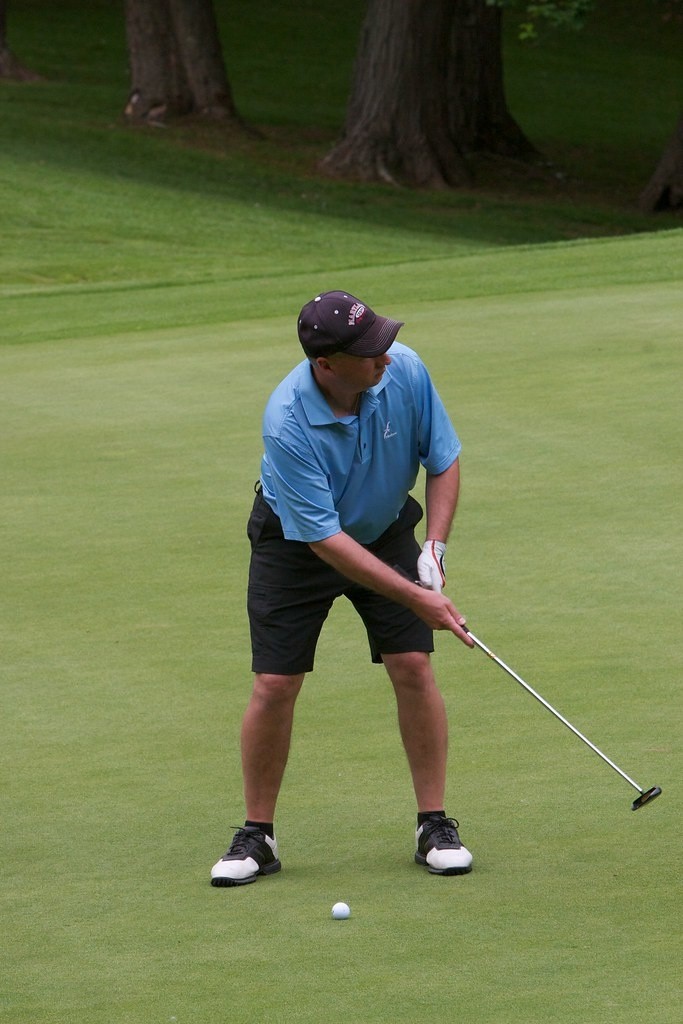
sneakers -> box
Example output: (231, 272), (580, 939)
(210, 826), (281, 886)
(415, 814), (473, 873)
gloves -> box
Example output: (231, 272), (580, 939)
(417, 540), (446, 593)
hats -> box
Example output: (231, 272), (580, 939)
(298, 290), (406, 356)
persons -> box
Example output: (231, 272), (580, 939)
(209, 290), (473, 888)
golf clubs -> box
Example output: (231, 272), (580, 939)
(397, 567), (664, 811)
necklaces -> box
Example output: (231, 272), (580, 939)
(353, 393), (360, 417)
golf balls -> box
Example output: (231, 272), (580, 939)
(331, 901), (350, 919)
